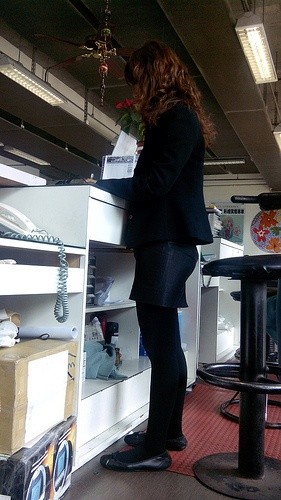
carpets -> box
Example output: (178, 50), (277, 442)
(120, 359), (281, 477)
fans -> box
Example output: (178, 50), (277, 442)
(35, 0), (136, 69)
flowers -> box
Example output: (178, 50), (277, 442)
(115, 98), (145, 141)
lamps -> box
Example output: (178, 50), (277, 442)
(236, 12), (278, 84)
(0, 54), (67, 107)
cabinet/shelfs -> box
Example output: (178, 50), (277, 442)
(0, 184), (244, 472)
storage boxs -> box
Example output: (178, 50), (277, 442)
(0, 338), (78, 456)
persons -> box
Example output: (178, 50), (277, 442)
(83, 40), (214, 473)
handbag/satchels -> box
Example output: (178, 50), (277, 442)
(83, 339), (129, 380)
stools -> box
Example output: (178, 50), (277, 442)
(197, 192), (281, 500)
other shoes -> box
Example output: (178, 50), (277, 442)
(125, 430), (188, 450)
(101, 450), (173, 471)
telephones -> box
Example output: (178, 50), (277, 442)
(0, 201), (54, 241)
